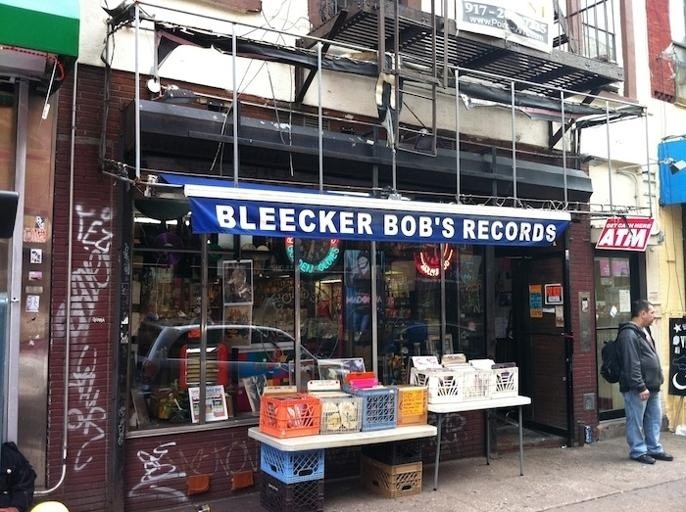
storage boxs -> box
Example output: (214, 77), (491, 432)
(255, 385), (429, 512)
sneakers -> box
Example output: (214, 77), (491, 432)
(650, 452), (674, 462)
(631, 455), (656, 464)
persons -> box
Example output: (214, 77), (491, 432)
(348, 253), (371, 342)
(615, 300), (673, 464)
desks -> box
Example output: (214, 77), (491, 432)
(248, 395), (531, 492)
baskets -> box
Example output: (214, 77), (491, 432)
(259, 365), (519, 440)
(361, 440), (424, 499)
(260, 443), (326, 512)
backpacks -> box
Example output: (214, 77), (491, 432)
(599, 324), (641, 384)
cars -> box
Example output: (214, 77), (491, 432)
(136, 324), (319, 399)
(377, 323), (476, 384)
